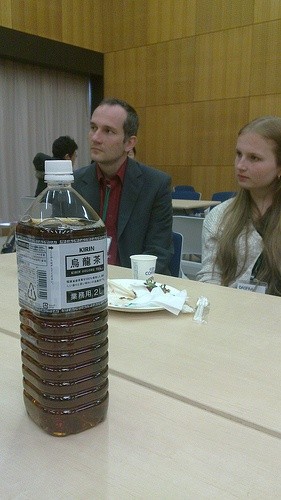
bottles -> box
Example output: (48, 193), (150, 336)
(14, 160), (109, 437)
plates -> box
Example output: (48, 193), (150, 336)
(107, 278), (179, 313)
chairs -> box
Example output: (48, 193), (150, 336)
(172, 186), (237, 279)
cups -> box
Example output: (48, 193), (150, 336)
(130, 254), (157, 279)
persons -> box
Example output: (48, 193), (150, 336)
(128, 147), (136, 160)
(33, 136), (79, 197)
(52, 97), (175, 276)
(196, 115), (281, 298)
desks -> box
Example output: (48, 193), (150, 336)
(171, 198), (221, 211)
(0, 248), (280, 500)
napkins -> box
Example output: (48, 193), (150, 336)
(133, 289), (187, 315)
